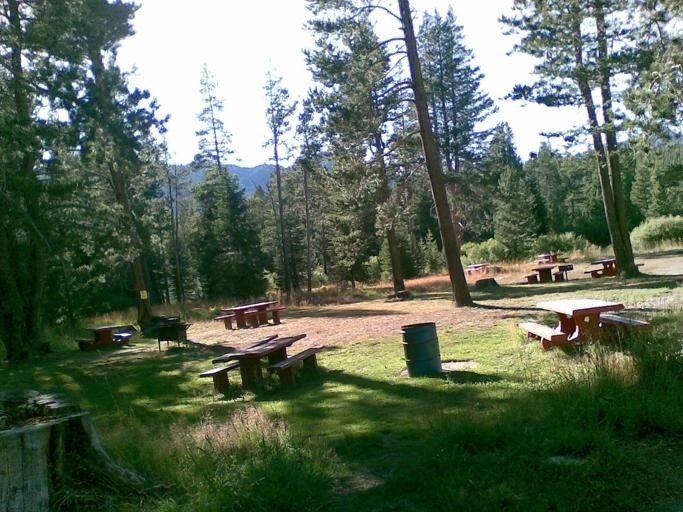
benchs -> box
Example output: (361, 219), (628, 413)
(76, 301), (326, 391)
(465, 252), (652, 352)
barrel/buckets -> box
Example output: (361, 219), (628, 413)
(401, 323), (442, 377)
